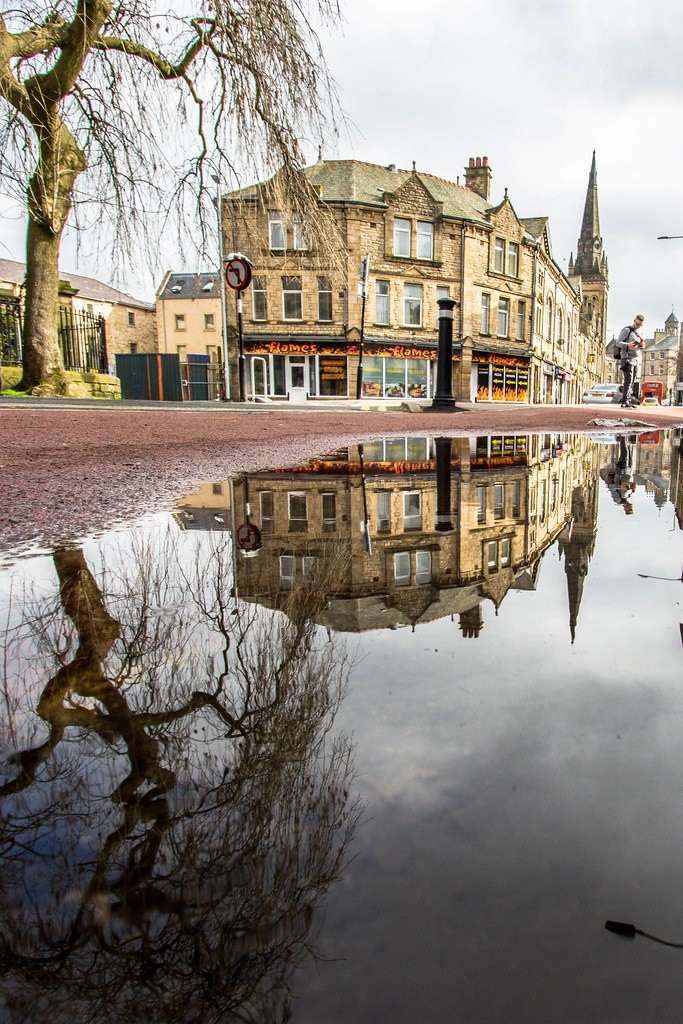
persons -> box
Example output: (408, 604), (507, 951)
(616, 315), (645, 408)
(608, 435), (637, 515)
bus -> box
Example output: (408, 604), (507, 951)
(640, 381), (663, 405)
(639, 430), (661, 447)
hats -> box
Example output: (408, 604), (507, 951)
(636, 315), (644, 321)
(626, 511), (633, 514)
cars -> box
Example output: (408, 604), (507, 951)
(583, 384), (632, 404)
(641, 398), (660, 406)
(584, 433), (620, 445)
(631, 395), (641, 405)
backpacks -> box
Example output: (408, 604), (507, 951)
(613, 326), (632, 359)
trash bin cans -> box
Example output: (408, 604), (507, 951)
(288, 387), (308, 402)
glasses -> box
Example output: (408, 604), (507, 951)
(635, 321), (642, 326)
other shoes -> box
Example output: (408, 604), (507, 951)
(620, 403), (638, 409)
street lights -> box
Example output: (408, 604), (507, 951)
(209, 174), (232, 402)
